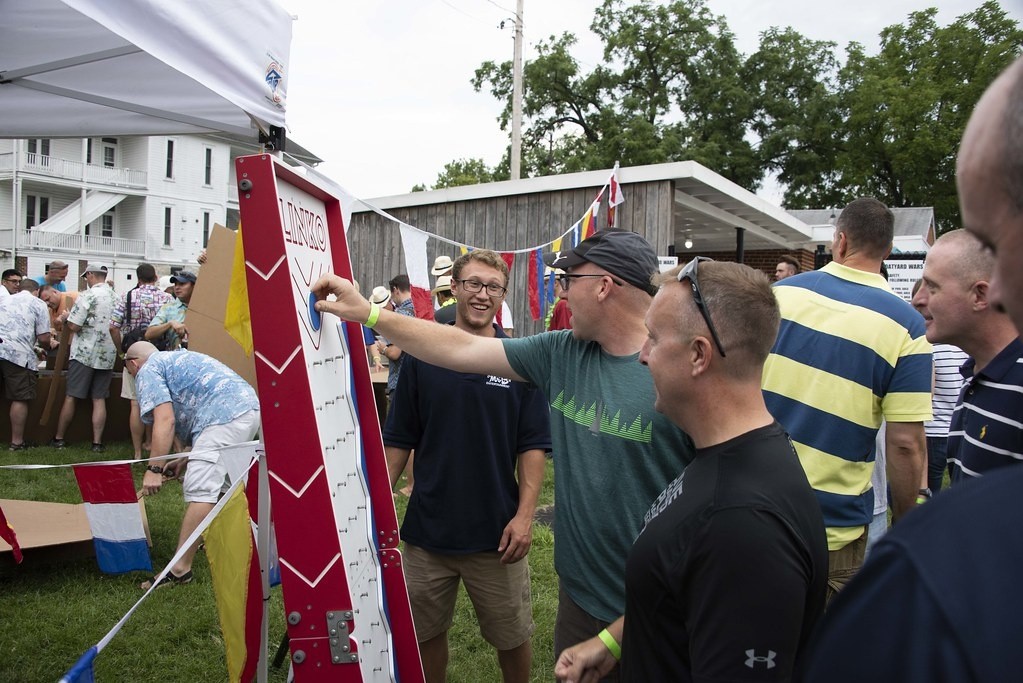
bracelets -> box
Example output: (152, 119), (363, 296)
(373, 357), (381, 361)
(382, 347), (387, 355)
(598, 628), (621, 660)
(364, 302), (379, 328)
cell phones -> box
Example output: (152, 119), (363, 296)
(375, 336), (385, 342)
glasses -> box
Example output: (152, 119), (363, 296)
(51, 264), (68, 269)
(455, 279), (506, 298)
(121, 357), (139, 367)
(4, 278), (21, 283)
(179, 272), (196, 280)
(560, 274), (622, 291)
(677, 256), (727, 359)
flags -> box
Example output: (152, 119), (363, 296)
(72, 463), (152, 573)
(399, 223), (436, 321)
(460, 245), (515, 331)
(528, 173), (625, 321)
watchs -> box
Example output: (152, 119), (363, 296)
(919, 488), (932, 497)
(147, 464), (163, 473)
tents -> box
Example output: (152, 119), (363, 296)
(0, 0), (294, 683)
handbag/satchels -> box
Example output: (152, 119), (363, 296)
(122, 331), (166, 353)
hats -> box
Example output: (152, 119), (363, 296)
(543, 227), (660, 297)
(170, 270), (197, 283)
(431, 274), (452, 295)
(544, 264), (566, 280)
(431, 256), (455, 276)
(369, 286), (391, 308)
(80, 263), (109, 277)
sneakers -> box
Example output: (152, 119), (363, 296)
(46, 438), (65, 448)
(90, 443), (107, 453)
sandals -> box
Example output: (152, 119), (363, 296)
(197, 542), (207, 550)
(9, 440), (39, 451)
(140, 568), (194, 591)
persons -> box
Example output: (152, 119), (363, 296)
(354, 256), (514, 497)
(0, 254), (207, 464)
(553, 55), (1023, 683)
(125, 341), (261, 591)
(383, 249), (553, 683)
(311, 227), (695, 683)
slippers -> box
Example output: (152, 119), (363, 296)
(393, 487), (412, 499)
